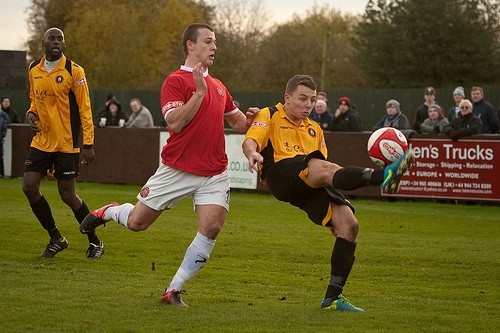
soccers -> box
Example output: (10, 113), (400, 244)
(366, 127), (409, 168)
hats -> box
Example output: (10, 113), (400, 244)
(386, 100), (400, 111)
(425, 87), (436, 96)
(338, 98), (351, 107)
(452, 87), (465, 98)
(429, 105), (441, 116)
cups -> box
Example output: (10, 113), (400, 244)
(101, 118), (107, 127)
(120, 119), (125, 127)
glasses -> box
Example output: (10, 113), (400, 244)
(460, 106), (471, 110)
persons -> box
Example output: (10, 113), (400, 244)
(224, 101), (240, 128)
(1, 97), (20, 179)
(92, 95), (116, 125)
(419, 104), (449, 135)
(79, 24), (260, 307)
(97, 100), (128, 127)
(471, 86), (499, 134)
(0, 101), (10, 179)
(125, 98), (153, 128)
(21, 28), (105, 260)
(445, 99), (481, 140)
(372, 99), (410, 132)
(412, 86), (439, 131)
(448, 86), (465, 122)
(242, 75), (413, 313)
(312, 92), (333, 131)
(333, 97), (361, 132)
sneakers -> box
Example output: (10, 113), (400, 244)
(40, 237), (68, 258)
(78, 202), (120, 234)
(161, 287), (189, 308)
(379, 150), (413, 194)
(321, 293), (364, 313)
(86, 241), (105, 258)
